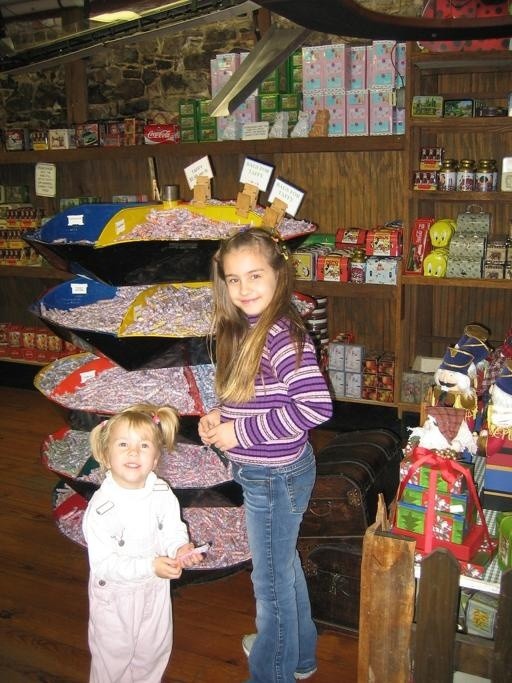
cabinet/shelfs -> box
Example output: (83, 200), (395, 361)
(0, 47), (406, 415)
(403, 41), (512, 409)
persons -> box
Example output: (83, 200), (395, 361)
(195, 222), (338, 682)
(79, 401), (206, 681)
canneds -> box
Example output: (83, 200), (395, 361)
(3, 128), (24, 151)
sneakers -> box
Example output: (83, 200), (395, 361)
(241, 631), (318, 680)
